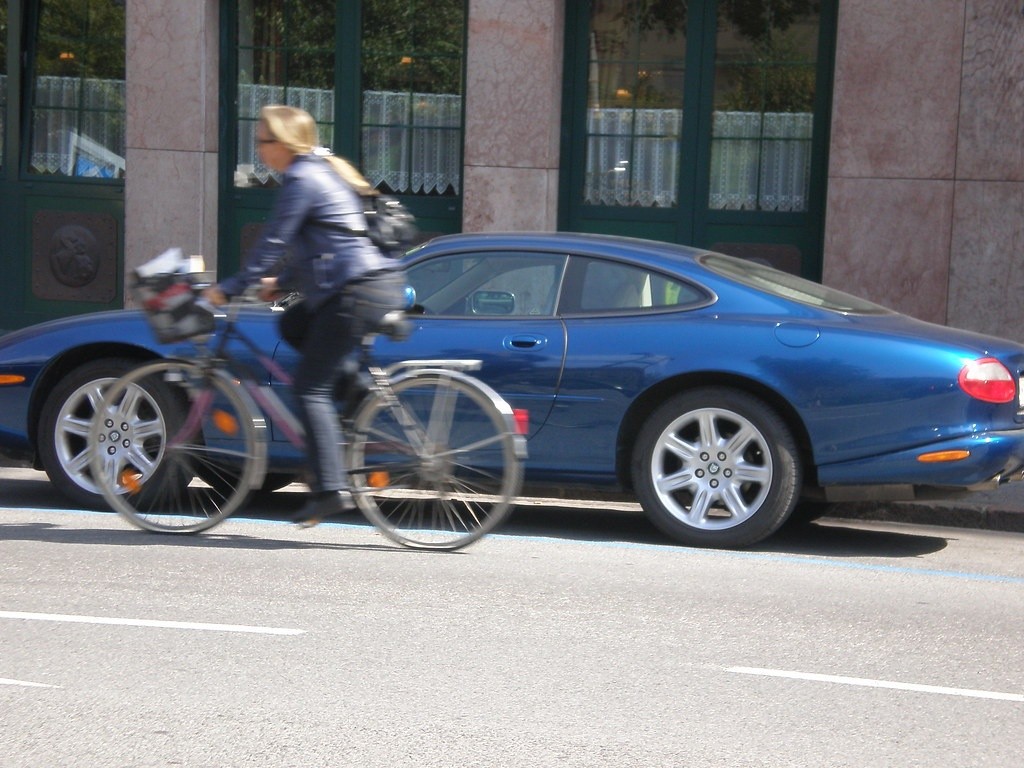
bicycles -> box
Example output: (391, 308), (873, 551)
(88, 271), (532, 552)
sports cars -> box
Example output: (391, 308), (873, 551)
(0, 231), (1024, 551)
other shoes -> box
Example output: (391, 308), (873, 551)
(292, 488), (354, 522)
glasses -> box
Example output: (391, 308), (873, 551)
(252, 134), (277, 146)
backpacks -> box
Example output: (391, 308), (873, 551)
(281, 156), (418, 255)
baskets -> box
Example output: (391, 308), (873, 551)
(129, 263), (217, 343)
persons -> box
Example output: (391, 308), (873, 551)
(199, 104), (408, 523)
